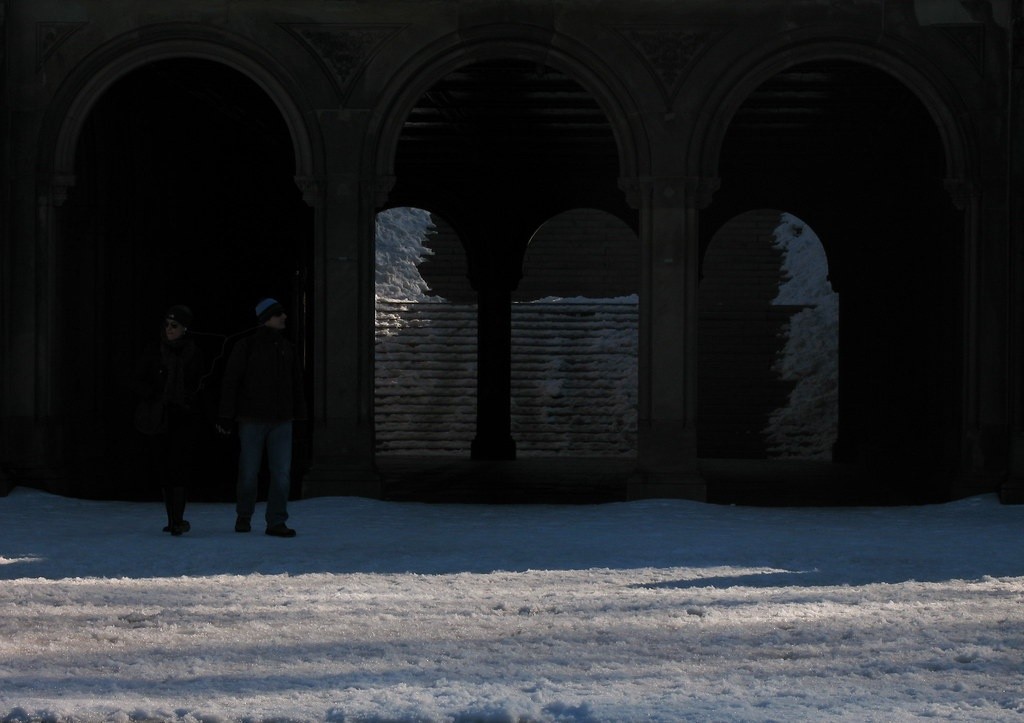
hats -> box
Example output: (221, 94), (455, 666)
(166, 305), (191, 326)
(255, 298), (282, 321)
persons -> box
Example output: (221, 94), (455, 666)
(216, 299), (309, 538)
(134, 305), (215, 536)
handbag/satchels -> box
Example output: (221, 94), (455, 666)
(135, 397), (169, 434)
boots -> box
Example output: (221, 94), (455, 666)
(163, 487), (189, 535)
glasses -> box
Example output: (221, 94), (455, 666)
(163, 323), (177, 329)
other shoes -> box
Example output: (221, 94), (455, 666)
(265, 523), (296, 537)
(235, 516), (250, 532)
